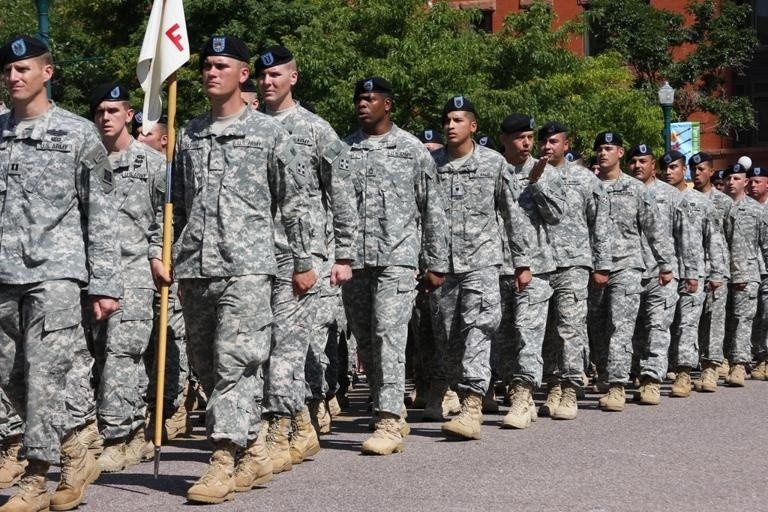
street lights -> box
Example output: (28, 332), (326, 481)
(735, 155), (752, 177)
(657, 81), (674, 158)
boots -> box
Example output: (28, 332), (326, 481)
(185, 436), (239, 506)
(500, 379), (539, 430)
(539, 354), (767, 441)
(441, 391), (485, 442)
(49, 427), (102, 512)
(405, 376), (462, 426)
(266, 383), (352, 476)
(234, 420), (274, 494)
(483, 378), (500, 415)
(359, 392), (412, 456)
(0, 458), (51, 512)
(0, 373), (212, 490)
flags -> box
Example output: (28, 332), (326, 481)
(137, 1), (191, 137)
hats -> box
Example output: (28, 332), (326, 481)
(0, 34), (50, 67)
(87, 80), (131, 112)
(589, 128), (768, 183)
(414, 125), (445, 146)
(352, 76), (393, 101)
(473, 134), (499, 153)
(442, 95), (479, 122)
(237, 78), (259, 94)
(536, 120), (568, 143)
(499, 112), (536, 137)
(131, 110), (171, 133)
(253, 45), (295, 73)
(562, 146), (584, 164)
(199, 34), (251, 75)
